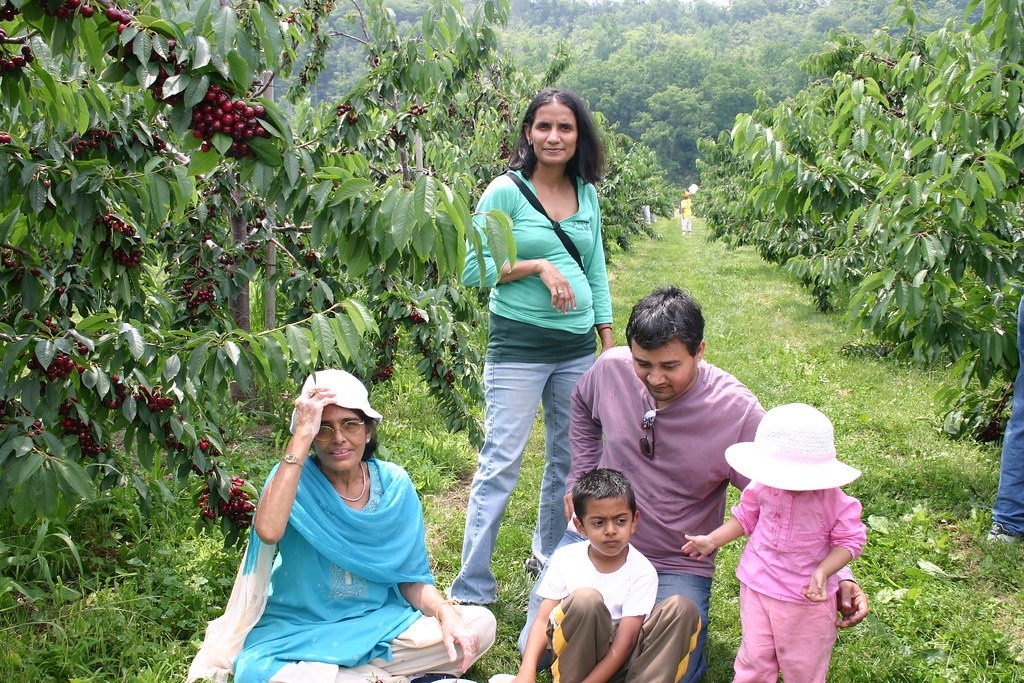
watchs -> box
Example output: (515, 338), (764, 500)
(282, 454), (304, 467)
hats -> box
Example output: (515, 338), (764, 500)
(290, 368), (383, 436)
(725, 403), (863, 491)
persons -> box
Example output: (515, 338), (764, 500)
(190, 369), (497, 683)
(680, 191), (692, 236)
(681, 403), (867, 683)
(511, 469), (699, 683)
(519, 285), (867, 683)
(642, 205), (656, 226)
(987, 295), (1024, 543)
(447, 88), (611, 605)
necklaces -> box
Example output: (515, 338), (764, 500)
(336, 463), (366, 502)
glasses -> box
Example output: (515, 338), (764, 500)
(640, 410), (657, 460)
(315, 420), (366, 441)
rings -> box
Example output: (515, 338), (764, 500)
(557, 290), (564, 294)
(309, 389), (317, 397)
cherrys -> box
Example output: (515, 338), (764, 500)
(0, 0), (513, 535)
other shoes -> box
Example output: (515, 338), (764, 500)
(527, 556), (542, 580)
(411, 672), (476, 683)
(987, 523), (1018, 544)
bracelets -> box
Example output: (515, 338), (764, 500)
(598, 327), (612, 334)
(435, 600), (463, 624)
